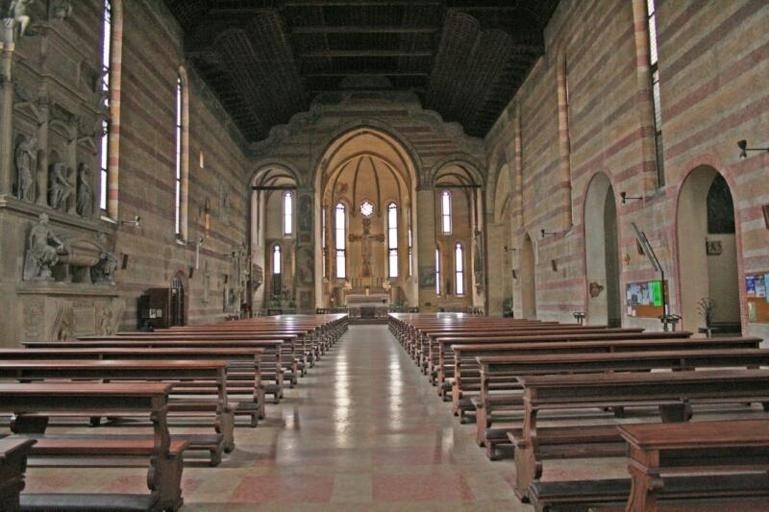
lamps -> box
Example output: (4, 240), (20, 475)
(620, 191), (642, 204)
(122, 216), (142, 228)
(504, 247), (515, 254)
(737, 140), (769, 159)
(541, 229), (556, 238)
